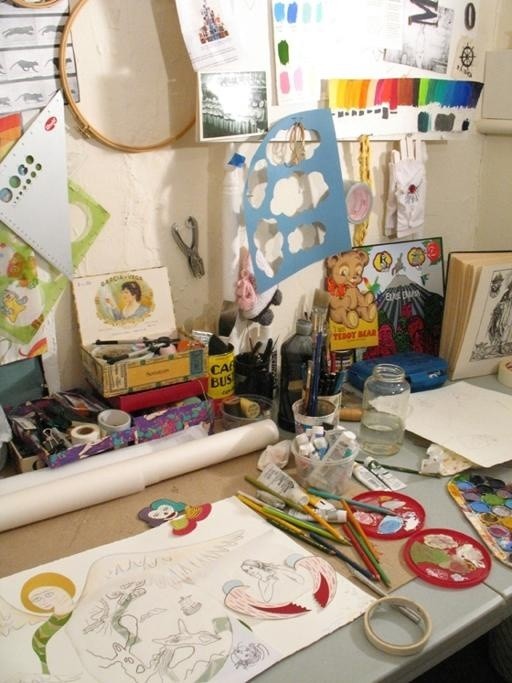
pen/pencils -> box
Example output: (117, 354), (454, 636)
(236, 474), (421, 625)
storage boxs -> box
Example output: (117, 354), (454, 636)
(347, 351), (448, 395)
(72, 331), (204, 399)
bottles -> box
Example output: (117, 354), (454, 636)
(276, 316), (312, 432)
(358, 361), (410, 457)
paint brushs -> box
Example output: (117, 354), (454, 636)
(301, 308), (346, 417)
(354, 460), (441, 479)
(248, 336), (273, 364)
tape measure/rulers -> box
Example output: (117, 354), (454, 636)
(0, 87), (77, 282)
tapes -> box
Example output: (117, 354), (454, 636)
(70, 423), (101, 446)
(343, 180), (373, 223)
(363, 596), (432, 656)
(97, 408), (132, 434)
(496, 358), (512, 388)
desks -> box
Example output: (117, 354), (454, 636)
(0, 385), (508, 683)
(272, 338), (512, 623)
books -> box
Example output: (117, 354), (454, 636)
(438, 247), (512, 384)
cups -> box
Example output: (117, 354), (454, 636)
(231, 351), (274, 408)
(319, 387), (342, 425)
(94, 407), (134, 439)
(291, 396), (335, 437)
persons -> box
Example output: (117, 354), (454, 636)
(240, 557), (307, 605)
(104, 280), (149, 322)
(19, 570), (77, 615)
(234, 641), (271, 672)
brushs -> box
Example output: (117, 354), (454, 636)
(218, 299), (241, 344)
(209, 333), (226, 356)
(309, 288), (333, 334)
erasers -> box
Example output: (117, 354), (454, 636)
(256, 489), (286, 510)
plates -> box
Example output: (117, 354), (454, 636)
(404, 529), (491, 587)
(348, 489), (424, 539)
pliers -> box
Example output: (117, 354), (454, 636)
(171, 216), (205, 280)
